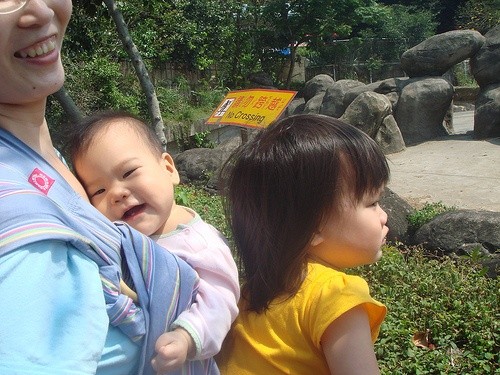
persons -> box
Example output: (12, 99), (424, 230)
(66, 112), (241, 375)
(0, 0), (219, 375)
(213, 114), (392, 375)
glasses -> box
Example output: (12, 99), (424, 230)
(0, 0), (28, 15)
